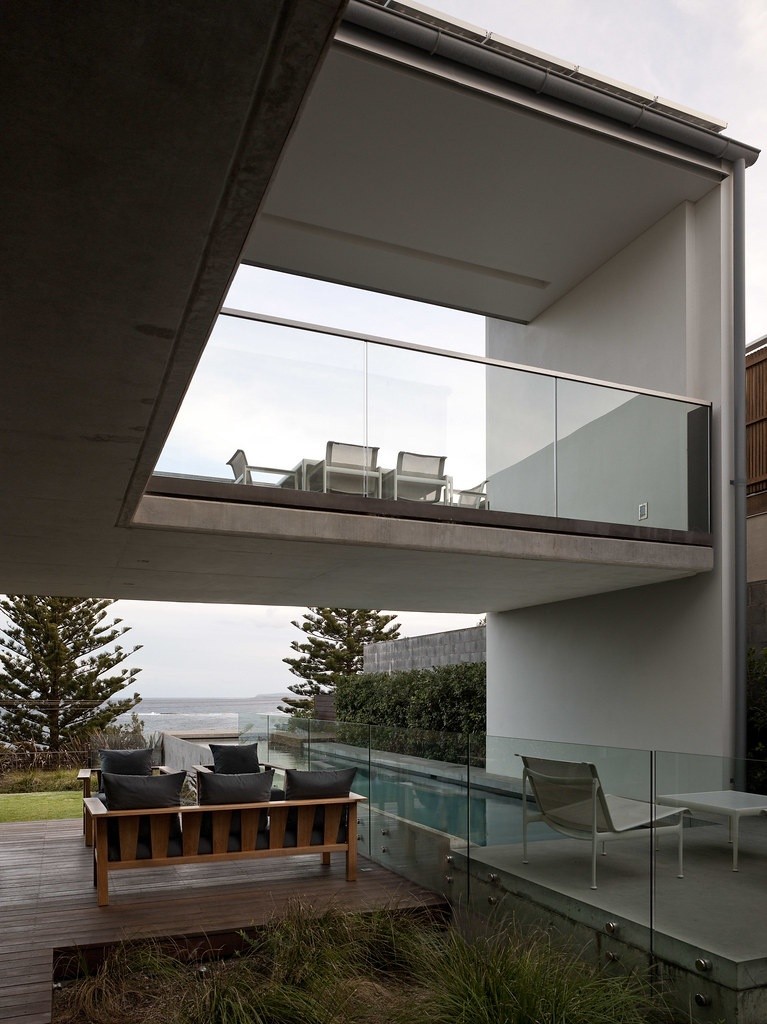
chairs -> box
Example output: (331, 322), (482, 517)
(307, 441), (383, 499)
(382, 451), (449, 506)
(513, 752), (693, 890)
(443, 475), (489, 511)
(190, 763), (297, 775)
(76, 766), (177, 846)
(226, 449), (298, 489)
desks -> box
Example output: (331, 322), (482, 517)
(657, 789), (767, 873)
(276, 458), (454, 504)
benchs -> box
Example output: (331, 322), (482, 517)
(84, 791), (367, 907)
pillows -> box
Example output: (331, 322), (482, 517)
(101, 771), (187, 843)
(98, 749), (153, 793)
(208, 742), (261, 773)
(197, 770), (274, 835)
(285, 767), (358, 829)
